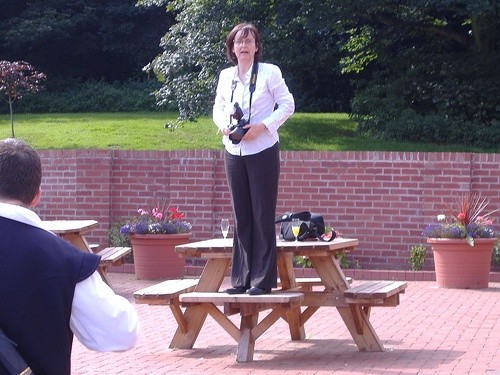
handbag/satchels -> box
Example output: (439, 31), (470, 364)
(275, 210), (337, 242)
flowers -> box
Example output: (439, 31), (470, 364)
(120, 198), (193, 235)
(421, 191), (499, 237)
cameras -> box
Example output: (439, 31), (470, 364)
(228, 102), (250, 144)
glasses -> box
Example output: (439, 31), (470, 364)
(232, 39), (256, 45)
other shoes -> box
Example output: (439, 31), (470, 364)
(245, 285), (272, 295)
(225, 286), (246, 294)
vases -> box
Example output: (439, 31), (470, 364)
(130, 234), (189, 282)
(427, 237), (498, 289)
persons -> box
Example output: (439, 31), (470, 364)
(212, 21), (295, 295)
(0, 137), (138, 375)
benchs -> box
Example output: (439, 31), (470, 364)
(96, 247), (132, 261)
(133, 277), (408, 305)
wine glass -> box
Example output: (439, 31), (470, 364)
(221, 219), (229, 241)
(292, 219), (300, 243)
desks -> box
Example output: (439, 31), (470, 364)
(167, 239), (386, 363)
(38, 218), (117, 297)
